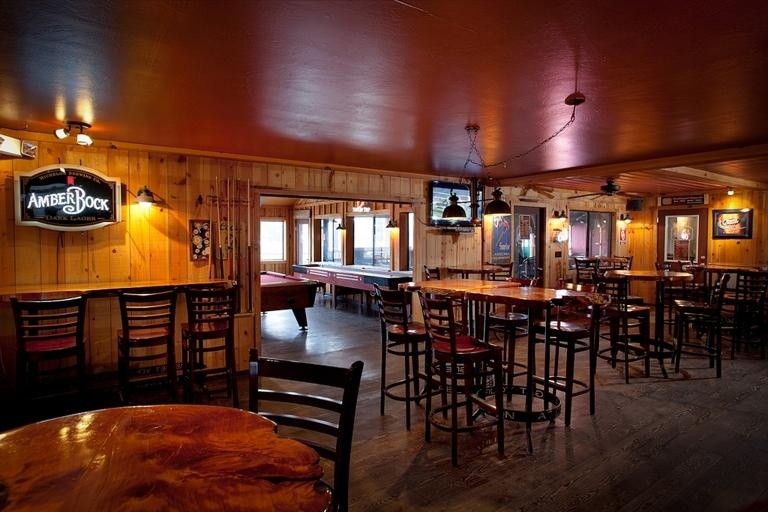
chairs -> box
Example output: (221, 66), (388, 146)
(536, 282), (602, 424)
(592, 275), (649, 384)
(116, 289), (177, 401)
(486, 261), (514, 281)
(671, 273), (729, 378)
(423, 265), (440, 281)
(179, 283), (240, 406)
(416, 289), (507, 466)
(9, 294), (86, 405)
(575, 254), (645, 303)
(374, 284), (450, 432)
(245, 348), (364, 512)
(487, 278), (536, 403)
(733, 269), (767, 362)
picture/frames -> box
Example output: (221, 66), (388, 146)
(712, 208), (753, 240)
(188, 218), (215, 264)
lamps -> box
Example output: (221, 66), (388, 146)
(552, 210), (567, 221)
(52, 121), (93, 147)
(484, 190), (512, 218)
(385, 219), (398, 233)
(620, 213), (632, 222)
(727, 187), (735, 196)
(136, 188), (154, 208)
(352, 201), (369, 213)
(335, 222), (345, 232)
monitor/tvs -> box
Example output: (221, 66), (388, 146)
(426, 179), (486, 226)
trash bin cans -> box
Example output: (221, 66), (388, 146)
(324, 294), (333, 309)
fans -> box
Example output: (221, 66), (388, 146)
(519, 184), (555, 200)
(565, 178), (645, 200)
